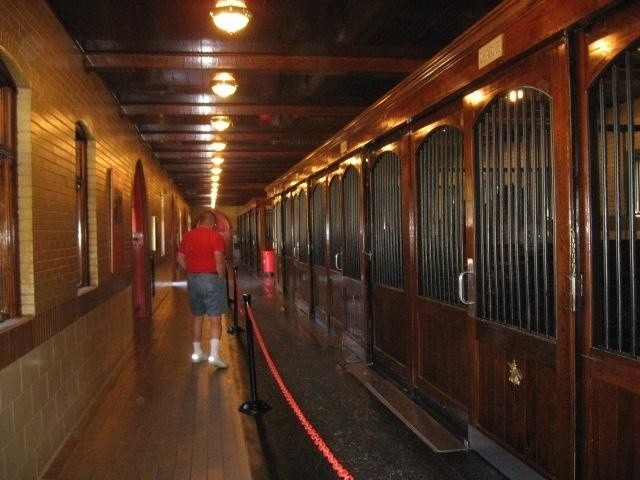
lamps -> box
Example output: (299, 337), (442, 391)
(210, 115), (232, 166)
(207, 0), (253, 36)
(211, 71), (238, 98)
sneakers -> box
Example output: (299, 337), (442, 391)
(207, 355), (229, 369)
(191, 350), (210, 364)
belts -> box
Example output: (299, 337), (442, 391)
(188, 271), (218, 276)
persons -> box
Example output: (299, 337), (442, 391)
(177, 211), (229, 368)
